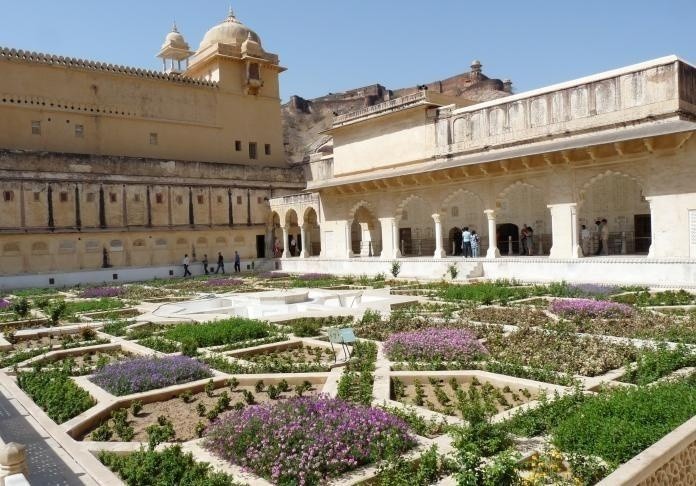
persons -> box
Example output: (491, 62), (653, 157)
(523, 226), (534, 255)
(599, 218), (610, 255)
(592, 220), (603, 255)
(201, 253), (210, 274)
(521, 223), (528, 254)
(233, 250), (240, 273)
(274, 236), (280, 257)
(214, 251), (225, 274)
(290, 237), (297, 256)
(469, 229), (480, 257)
(581, 224), (590, 256)
(182, 254), (191, 277)
(461, 226), (472, 256)
